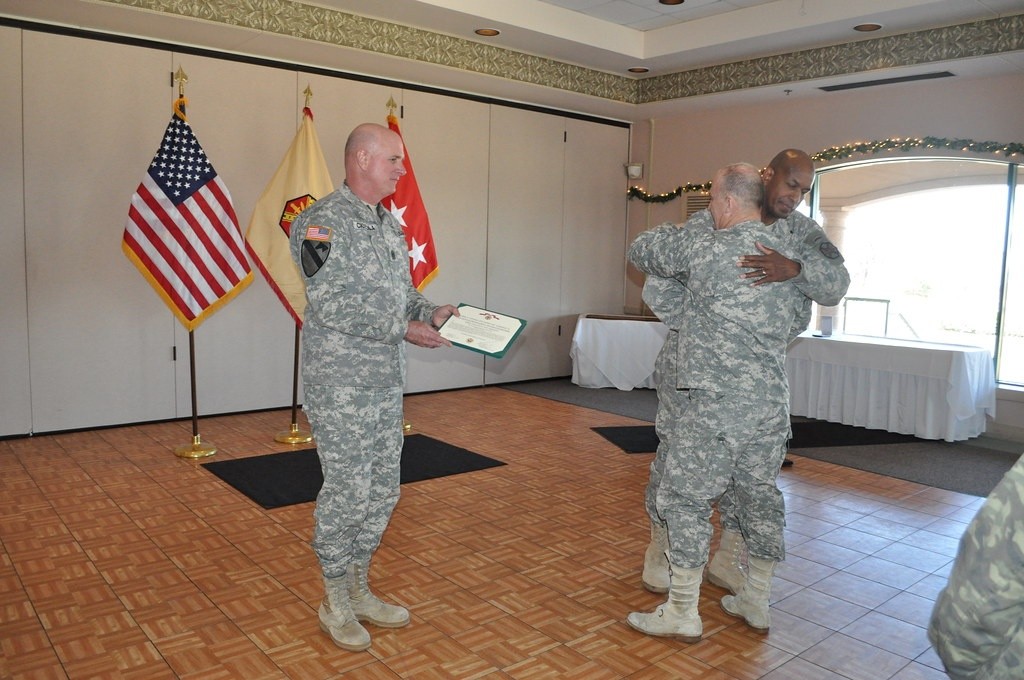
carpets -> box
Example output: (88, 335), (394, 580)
(588, 424), (793, 465)
(200, 433), (508, 511)
(497, 365), (1020, 497)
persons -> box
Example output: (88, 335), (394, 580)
(641, 147), (851, 595)
(925, 452), (1023, 680)
(625, 148), (851, 643)
(289, 122), (460, 654)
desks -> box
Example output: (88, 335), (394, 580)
(569, 311), (998, 444)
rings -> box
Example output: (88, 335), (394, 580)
(763, 269), (766, 275)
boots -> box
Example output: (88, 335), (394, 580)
(641, 521), (671, 594)
(317, 571), (372, 652)
(720, 552), (778, 634)
(708, 529), (749, 597)
(626, 549), (707, 643)
(347, 562), (411, 628)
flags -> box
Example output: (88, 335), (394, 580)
(244, 107), (334, 330)
(381, 115), (440, 295)
(120, 95), (256, 331)
